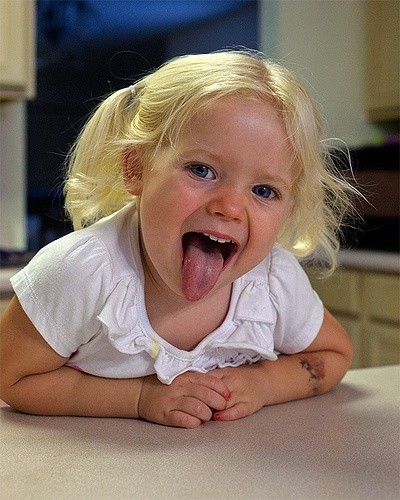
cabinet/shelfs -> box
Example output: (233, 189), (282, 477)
(360, 0), (399, 125)
(1, 1), (39, 104)
(287, 249), (400, 374)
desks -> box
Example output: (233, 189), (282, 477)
(1, 362), (400, 498)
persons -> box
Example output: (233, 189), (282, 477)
(0, 44), (371, 431)
